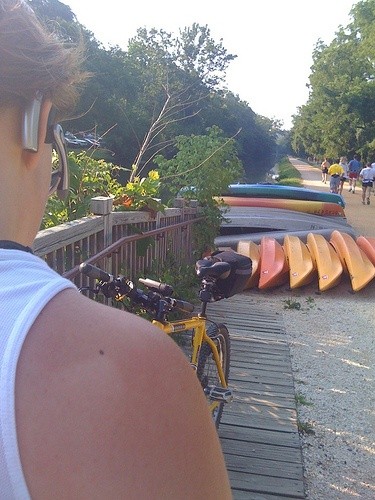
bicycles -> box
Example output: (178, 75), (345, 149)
(75, 249), (253, 431)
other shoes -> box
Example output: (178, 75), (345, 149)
(352, 191), (355, 194)
(322, 179), (324, 183)
(367, 198), (370, 205)
(325, 182), (326, 184)
(361, 202), (365, 205)
(348, 188), (352, 192)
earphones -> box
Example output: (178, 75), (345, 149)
(44, 105), (56, 143)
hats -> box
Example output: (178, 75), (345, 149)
(334, 160), (339, 162)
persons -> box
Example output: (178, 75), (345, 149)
(320, 158), (329, 184)
(371, 161), (375, 197)
(347, 154), (362, 193)
(337, 157), (349, 194)
(328, 159), (344, 194)
(359, 162), (374, 205)
(0, 0), (232, 500)
